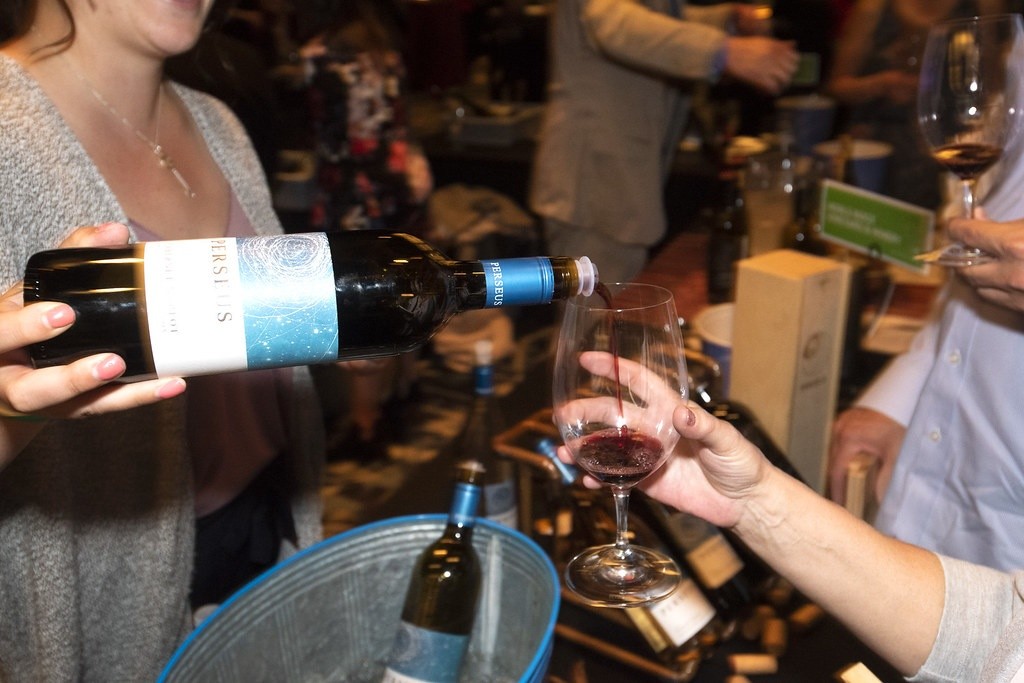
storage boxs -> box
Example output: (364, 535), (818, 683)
(726, 250), (853, 499)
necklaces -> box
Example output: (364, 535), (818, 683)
(27, 24), (197, 200)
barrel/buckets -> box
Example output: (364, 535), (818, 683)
(150, 512), (562, 683)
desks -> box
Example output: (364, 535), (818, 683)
(357, 193), (939, 683)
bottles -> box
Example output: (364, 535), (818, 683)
(382, 458), (489, 683)
(449, 339), (521, 536)
(538, 433), (763, 661)
(22, 228), (602, 386)
(689, 386), (820, 512)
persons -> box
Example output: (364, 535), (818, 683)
(259, 2), (434, 468)
(553, 352), (1024, 683)
(524, 0), (802, 332)
(826, 1), (1024, 579)
(0, 0), (330, 681)
(827, 0), (1009, 214)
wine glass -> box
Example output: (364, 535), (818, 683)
(552, 280), (692, 610)
(915, 12), (1024, 268)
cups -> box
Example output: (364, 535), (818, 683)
(692, 300), (734, 399)
